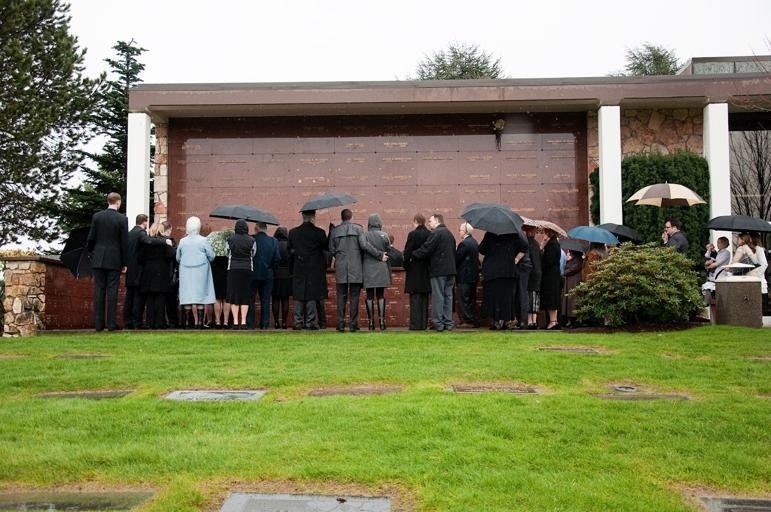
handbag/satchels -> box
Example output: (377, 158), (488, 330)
(732, 256), (762, 275)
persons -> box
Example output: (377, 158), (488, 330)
(660, 219), (689, 259)
(329, 208), (389, 332)
(704, 232), (768, 324)
(476, 224), (606, 332)
(124, 213), (177, 330)
(175, 217), (215, 329)
(361, 213), (391, 331)
(403, 214), (433, 329)
(204, 210), (331, 330)
(411, 214), (456, 331)
(85, 192), (128, 330)
(455, 222), (480, 328)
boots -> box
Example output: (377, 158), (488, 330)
(365, 299), (375, 330)
(566, 318), (576, 327)
(377, 299), (385, 330)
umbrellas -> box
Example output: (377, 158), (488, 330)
(625, 181), (707, 239)
(297, 191), (358, 225)
(460, 200), (643, 245)
(59, 225), (95, 281)
(208, 201), (280, 226)
(703, 214), (771, 234)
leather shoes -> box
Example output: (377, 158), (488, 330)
(195, 322), (286, 329)
(457, 324), (477, 328)
(336, 327), (360, 330)
(109, 324), (150, 330)
(490, 321), (562, 329)
(430, 326), (445, 332)
